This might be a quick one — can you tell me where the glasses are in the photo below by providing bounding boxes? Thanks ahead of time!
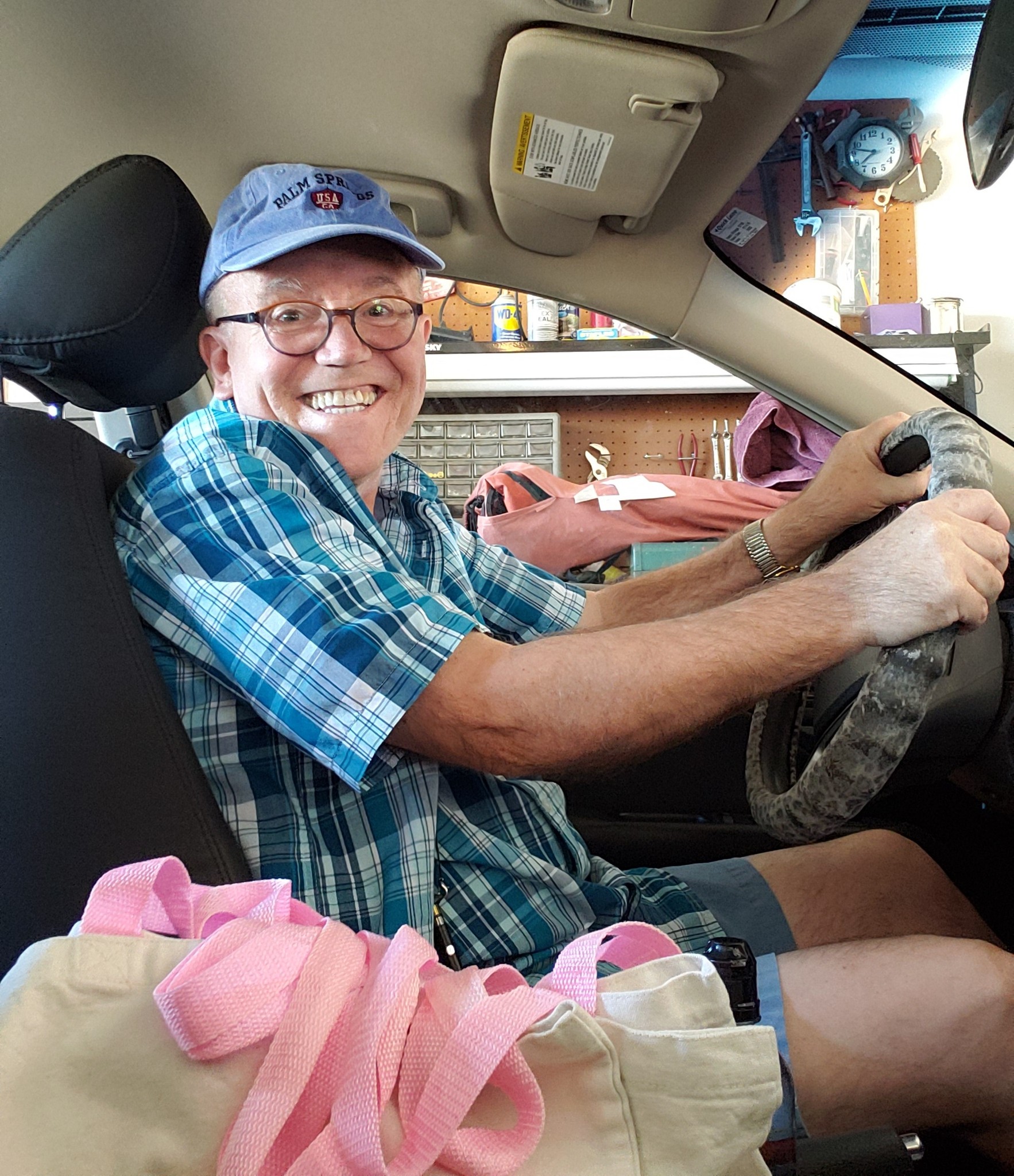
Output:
[214,295,424,357]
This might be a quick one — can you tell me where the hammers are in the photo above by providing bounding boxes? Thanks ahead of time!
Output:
[794,110,839,202]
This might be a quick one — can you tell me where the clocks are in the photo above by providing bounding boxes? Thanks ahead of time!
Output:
[835,116,913,193]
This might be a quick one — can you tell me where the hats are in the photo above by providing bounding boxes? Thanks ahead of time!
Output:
[199,163,446,308]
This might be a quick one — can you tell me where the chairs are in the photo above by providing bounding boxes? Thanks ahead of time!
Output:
[0,155,250,980]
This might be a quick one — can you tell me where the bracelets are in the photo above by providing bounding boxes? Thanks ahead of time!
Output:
[744,518,801,585]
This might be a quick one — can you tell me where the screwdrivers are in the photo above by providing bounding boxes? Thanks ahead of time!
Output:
[910,134,927,194]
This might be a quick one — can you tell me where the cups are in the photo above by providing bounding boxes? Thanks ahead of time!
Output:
[782,278,841,329]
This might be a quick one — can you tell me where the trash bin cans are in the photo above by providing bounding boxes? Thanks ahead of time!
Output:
[631,542,727,579]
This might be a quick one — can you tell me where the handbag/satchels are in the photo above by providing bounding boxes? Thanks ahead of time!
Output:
[0,856,784,1175]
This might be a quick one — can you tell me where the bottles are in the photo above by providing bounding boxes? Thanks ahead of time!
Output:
[703,936,798,1175]
[527,293,613,343]
[490,288,521,342]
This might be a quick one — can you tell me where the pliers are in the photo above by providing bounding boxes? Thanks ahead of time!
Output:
[678,434,698,477]
[899,127,940,185]
[811,179,861,206]
[963,0,1013,189]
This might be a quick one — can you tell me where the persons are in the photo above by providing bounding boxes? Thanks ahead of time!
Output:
[110,159,1012,1175]
